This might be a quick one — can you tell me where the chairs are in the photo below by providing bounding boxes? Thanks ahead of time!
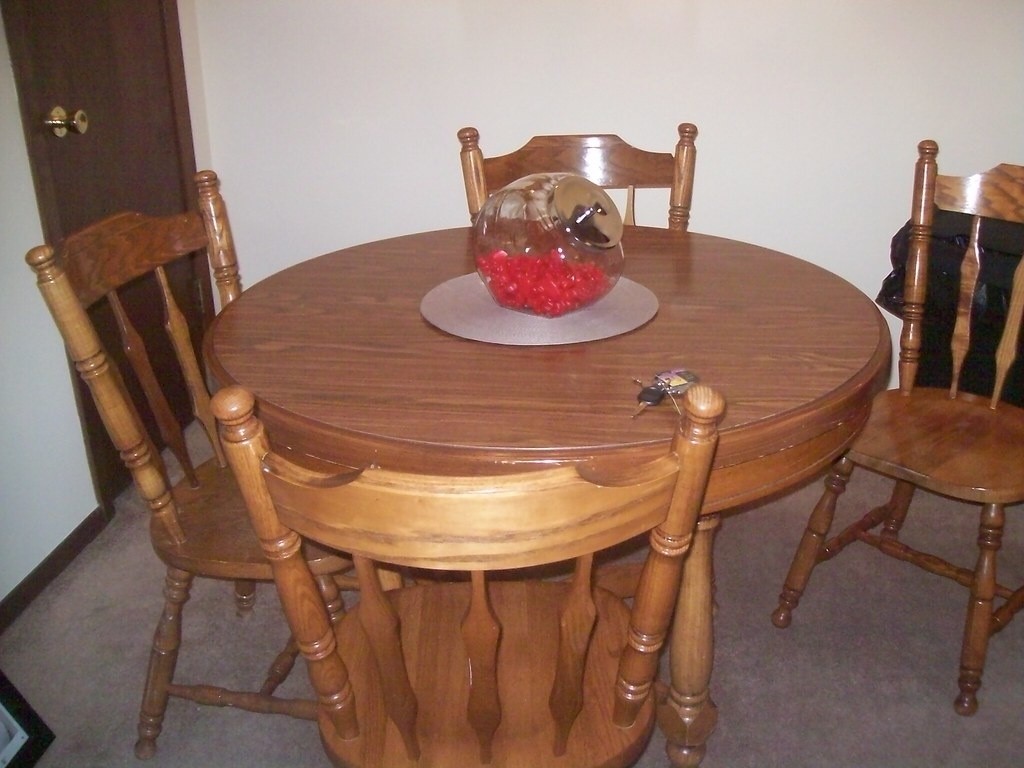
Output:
[770,140,1024,717]
[207,385,727,768]
[458,122,699,232]
[24,169,357,768]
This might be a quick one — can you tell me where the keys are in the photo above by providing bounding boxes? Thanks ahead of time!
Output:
[628,371,682,419]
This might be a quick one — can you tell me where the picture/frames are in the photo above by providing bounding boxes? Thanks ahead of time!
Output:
[0,669,56,768]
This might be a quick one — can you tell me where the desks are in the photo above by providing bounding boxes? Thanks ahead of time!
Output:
[199,225,892,598]
